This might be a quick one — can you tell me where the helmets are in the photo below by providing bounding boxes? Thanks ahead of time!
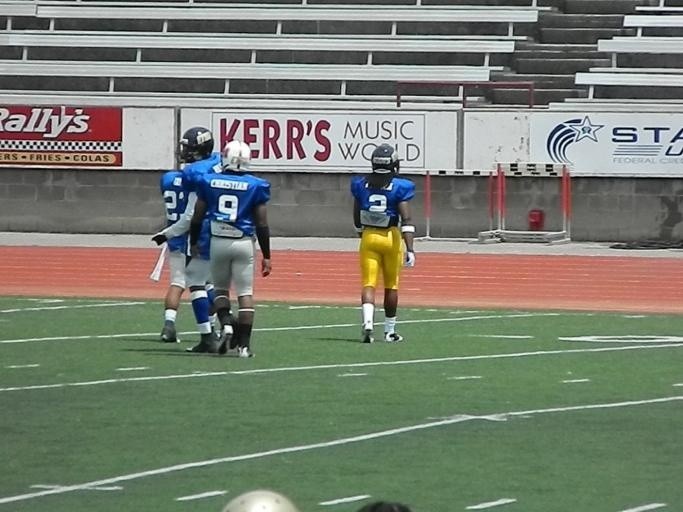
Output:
[371,144,399,176]
[221,141,251,173]
[180,127,213,161]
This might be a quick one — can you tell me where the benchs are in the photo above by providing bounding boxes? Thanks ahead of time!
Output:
[0,1,683,111]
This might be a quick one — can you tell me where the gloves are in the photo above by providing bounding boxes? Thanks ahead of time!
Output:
[405,251,415,267]
[151,232,167,245]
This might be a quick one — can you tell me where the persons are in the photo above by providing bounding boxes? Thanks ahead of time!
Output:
[150,126,241,352]
[190,141,271,359]
[159,170,217,338]
[349,143,416,344]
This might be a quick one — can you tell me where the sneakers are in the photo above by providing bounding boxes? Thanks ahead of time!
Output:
[161,320,255,358]
[385,332,403,342]
[362,321,375,343]
